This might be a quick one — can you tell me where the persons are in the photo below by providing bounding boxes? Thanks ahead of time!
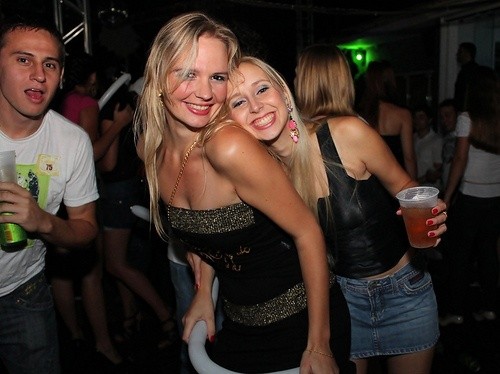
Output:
[50,52,178,374]
[225,45,448,374]
[439,98,463,194]
[453,42,481,113]
[411,105,444,199]
[353,60,418,184]
[132,13,357,374]
[0,18,100,374]
[440,66,500,327]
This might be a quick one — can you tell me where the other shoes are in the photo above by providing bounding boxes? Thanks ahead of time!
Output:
[440,316,464,327]
[472,311,497,323]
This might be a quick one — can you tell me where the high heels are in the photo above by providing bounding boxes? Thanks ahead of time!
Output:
[95,350,127,374]
[71,337,88,353]
[151,325,180,356]
[115,309,144,343]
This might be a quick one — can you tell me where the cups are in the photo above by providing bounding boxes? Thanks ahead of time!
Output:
[395,186,440,249]
[0,150,18,185]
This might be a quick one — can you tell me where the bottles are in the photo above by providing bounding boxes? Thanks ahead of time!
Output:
[0,201,28,253]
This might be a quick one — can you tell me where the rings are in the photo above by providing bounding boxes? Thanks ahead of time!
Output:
[442,211,448,217]
